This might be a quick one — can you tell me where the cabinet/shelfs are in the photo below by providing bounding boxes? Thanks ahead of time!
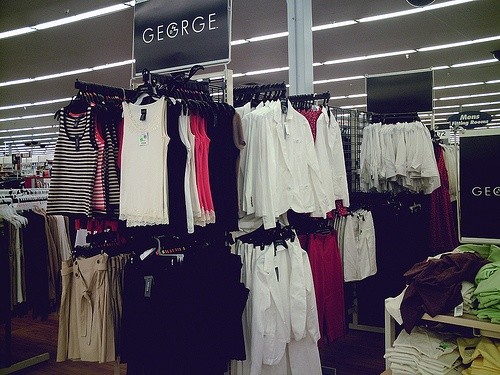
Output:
[382,296,500,375]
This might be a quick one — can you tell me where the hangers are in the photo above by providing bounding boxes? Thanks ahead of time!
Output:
[67,190,441,265]
[365,113,459,151]
[0,189,69,230]
[53,70,337,122]
